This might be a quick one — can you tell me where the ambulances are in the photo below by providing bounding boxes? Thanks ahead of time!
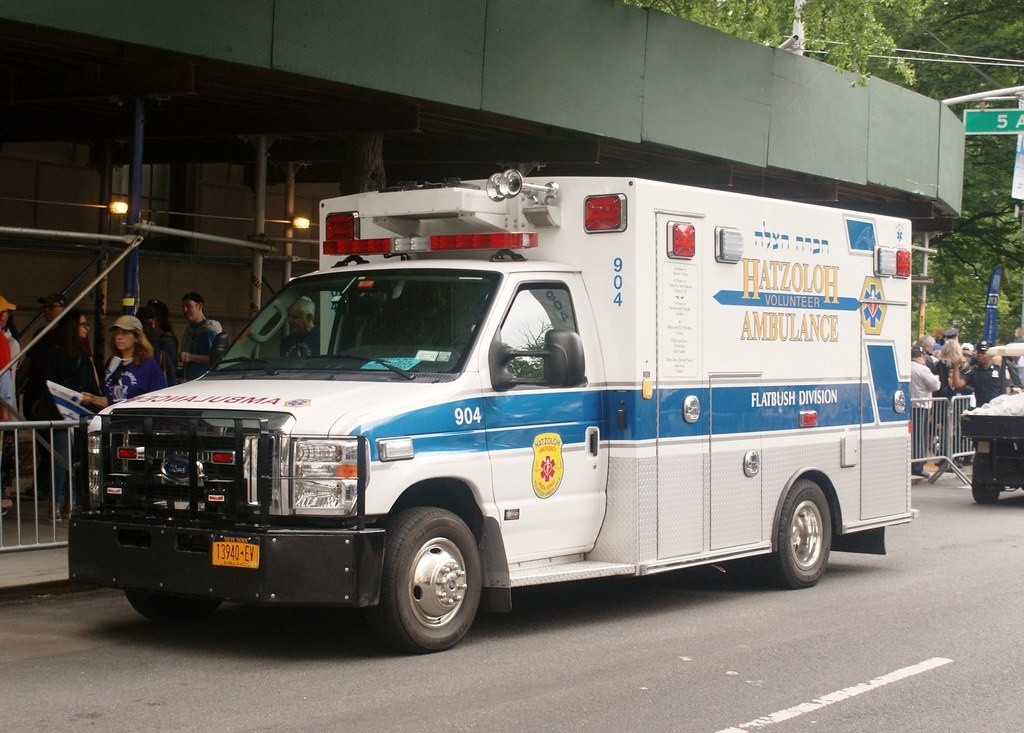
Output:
[66,167,920,661]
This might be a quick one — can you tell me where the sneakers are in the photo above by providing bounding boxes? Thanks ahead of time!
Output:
[24,487,49,499]
[2,496,9,516]
[62,511,69,519]
[48,513,62,523]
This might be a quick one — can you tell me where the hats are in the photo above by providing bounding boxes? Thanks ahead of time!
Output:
[944,329,959,337]
[0,295,16,314]
[183,292,204,304]
[977,341,992,350]
[935,328,943,335]
[37,294,66,306]
[108,315,143,332]
[962,343,974,350]
[911,345,926,355]
[147,299,168,320]
[932,344,941,350]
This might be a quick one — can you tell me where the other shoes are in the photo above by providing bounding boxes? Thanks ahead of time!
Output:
[912,474,929,485]
[935,456,972,471]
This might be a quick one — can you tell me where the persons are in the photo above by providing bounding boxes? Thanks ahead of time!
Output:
[278,295,321,358]
[908,324,1024,477]
[0,292,224,525]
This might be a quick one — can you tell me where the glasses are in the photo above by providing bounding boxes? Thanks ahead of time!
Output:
[79,322,89,326]
[40,305,63,310]
